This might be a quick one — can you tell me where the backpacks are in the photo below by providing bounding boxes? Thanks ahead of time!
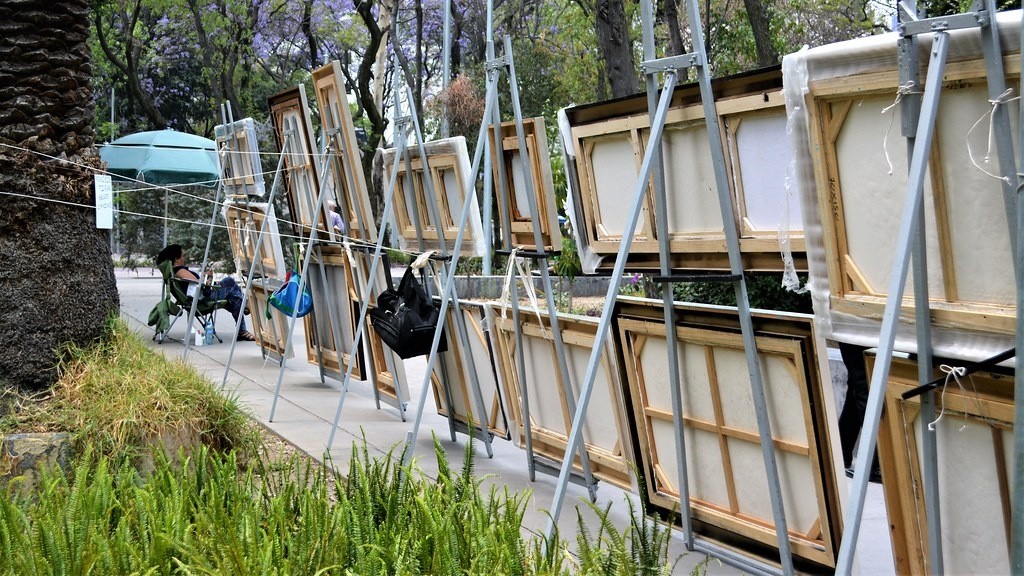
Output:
[370,253,448,360]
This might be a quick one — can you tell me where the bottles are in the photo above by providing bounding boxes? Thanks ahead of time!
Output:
[205,268,213,286]
[205,322,213,344]
[195,329,203,346]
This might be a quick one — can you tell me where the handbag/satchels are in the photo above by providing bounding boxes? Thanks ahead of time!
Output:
[265,243,314,320]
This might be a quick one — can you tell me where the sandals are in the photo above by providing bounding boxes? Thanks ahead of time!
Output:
[244,307,250,315]
[237,330,256,341]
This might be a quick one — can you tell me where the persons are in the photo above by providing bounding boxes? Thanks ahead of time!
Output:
[837,340,882,484]
[156,244,256,341]
[327,200,344,243]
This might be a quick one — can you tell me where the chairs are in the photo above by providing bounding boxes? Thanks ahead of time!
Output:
[157,260,226,344]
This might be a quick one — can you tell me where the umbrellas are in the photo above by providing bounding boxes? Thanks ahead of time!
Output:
[99,126,223,248]
